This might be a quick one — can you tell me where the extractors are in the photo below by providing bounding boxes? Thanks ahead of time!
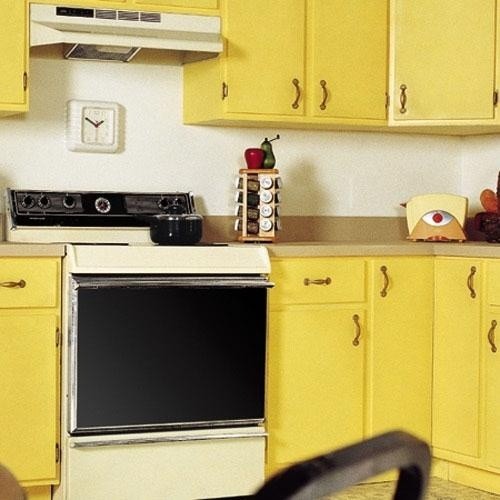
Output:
[29,3,225,64]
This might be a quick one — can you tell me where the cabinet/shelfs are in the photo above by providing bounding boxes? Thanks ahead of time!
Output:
[367,257,433,459]
[267,257,367,466]
[482,258,500,475]
[0,0,30,119]
[432,256,483,472]
[182,0,390,133]
[390,0,500,137]
[0,258,61,488]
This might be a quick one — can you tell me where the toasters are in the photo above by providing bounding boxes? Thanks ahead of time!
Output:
[400,193,469,242]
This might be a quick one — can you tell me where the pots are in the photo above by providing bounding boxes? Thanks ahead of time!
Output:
[146,205,204,243]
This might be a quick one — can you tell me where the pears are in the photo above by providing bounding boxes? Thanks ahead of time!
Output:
[260,134,280,169]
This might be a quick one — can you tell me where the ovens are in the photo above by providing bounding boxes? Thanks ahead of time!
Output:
[67,272,274,438]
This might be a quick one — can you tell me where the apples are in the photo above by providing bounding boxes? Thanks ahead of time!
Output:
[245,149,265,169]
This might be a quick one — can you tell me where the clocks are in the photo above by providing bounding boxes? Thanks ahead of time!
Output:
[67,99,121,153]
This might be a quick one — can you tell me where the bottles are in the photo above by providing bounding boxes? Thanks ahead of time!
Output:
[235,176,259,237]
